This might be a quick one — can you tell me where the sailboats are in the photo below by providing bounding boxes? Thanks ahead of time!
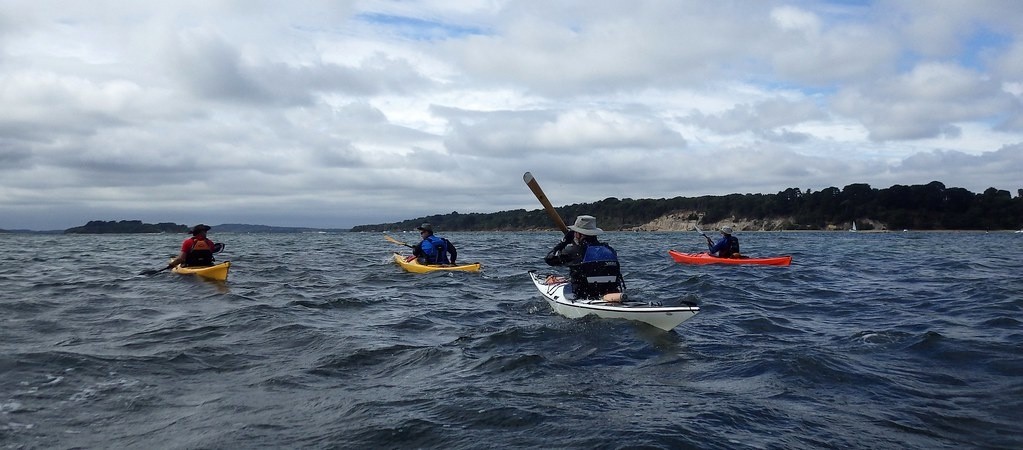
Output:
[849,221,857,233]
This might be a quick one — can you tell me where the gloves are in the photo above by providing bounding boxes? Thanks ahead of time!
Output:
[561,230,573,244]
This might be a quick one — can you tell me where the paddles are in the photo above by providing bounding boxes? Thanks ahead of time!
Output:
[384,235,416,249]
[139,242,225,277]
[522,172,577,245]
[693,224,716,245]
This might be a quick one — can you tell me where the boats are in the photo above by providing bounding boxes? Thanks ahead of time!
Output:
[393,251,480,273]
[168,258,230,281]
[529,272,701,332]
[668,250,792,267]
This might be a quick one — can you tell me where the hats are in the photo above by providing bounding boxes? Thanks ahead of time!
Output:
[718,226,733,234]
[567,215,604,236]
[189,225,211,234]
[417,223,431,231]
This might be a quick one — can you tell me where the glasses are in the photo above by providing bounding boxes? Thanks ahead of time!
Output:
[420,231,425,235]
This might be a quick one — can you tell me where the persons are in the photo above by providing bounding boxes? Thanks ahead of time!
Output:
[411,223,457,264]
[168,224,217,268]
[707,225,740,257]
[544,215,621,299]
